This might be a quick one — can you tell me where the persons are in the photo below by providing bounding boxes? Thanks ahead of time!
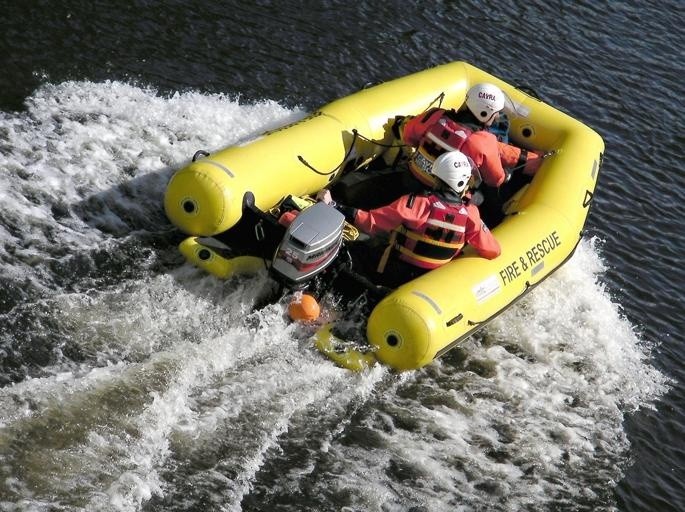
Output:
[316,151,502,285]
[400,83,518,197]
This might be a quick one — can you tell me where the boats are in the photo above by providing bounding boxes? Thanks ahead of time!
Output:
[164,59,606,375]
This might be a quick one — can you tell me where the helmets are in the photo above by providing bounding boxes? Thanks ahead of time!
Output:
[431,150,472,194]
[464,82,507,123]
